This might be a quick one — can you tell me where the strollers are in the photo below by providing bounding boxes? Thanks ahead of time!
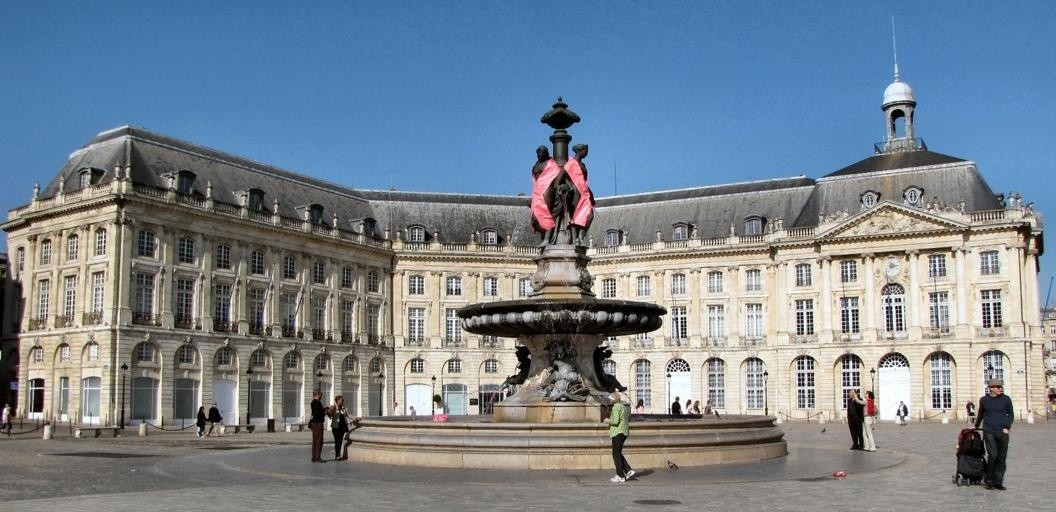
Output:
[951,428,987,485]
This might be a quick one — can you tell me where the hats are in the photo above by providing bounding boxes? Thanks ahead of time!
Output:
[608,392,620,400]
[986,378,1004,387]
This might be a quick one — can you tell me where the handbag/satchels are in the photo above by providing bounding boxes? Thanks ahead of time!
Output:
[956,428,987,455]
[307,415,314,429]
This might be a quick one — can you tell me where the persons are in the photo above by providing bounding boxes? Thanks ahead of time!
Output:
[969,379,1014,490]
[196,401,224,438]
[965,399,975,423]
[0,403,15,439]
[393,402,402,417]
[672,396,714,415]
[847,389,876,452]
[529,144,596,249]
[896,400,908,426]
[307,390,362,463]
[604,393,636,482]
[408,406,417,416]
[636,398,645,414]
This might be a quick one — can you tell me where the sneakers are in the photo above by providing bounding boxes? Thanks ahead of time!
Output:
[311,456,344,463]
[983,483,1007,491]
[626,470,637,481]
[609,474,627,483]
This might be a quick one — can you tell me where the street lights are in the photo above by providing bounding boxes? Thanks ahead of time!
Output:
[120,363,128,429]
[431,375,436,415]
[246,367,253,430]
[378,372,384,415]
[666,372,672,413]
[317,371,323,390]
[987,364,994,380]
[870,368,876,393]
[763,370,768,414]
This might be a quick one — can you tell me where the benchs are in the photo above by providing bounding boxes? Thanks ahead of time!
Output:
[286,422,308,432]
[74,426,121,439]
[220,424,255,433]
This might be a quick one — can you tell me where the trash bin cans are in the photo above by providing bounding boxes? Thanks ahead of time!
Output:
[268,419,274,432]
